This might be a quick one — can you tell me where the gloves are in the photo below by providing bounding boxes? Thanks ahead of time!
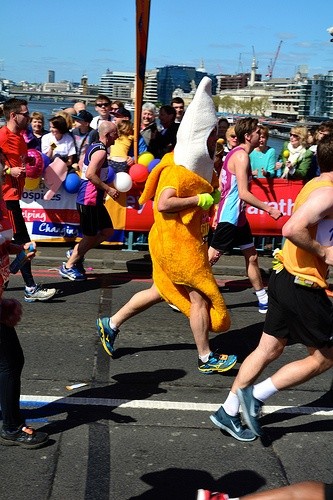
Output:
[211,189,221,204]
[197,193,214,211]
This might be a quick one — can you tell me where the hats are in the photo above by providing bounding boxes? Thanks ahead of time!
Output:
[72,110,93,123]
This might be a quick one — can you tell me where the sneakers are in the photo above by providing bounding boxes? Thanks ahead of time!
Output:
[65,249,93,271]
[258,301,270,313]
[25,282,57,302]
[198,349,238,375]
[0,423,50,449]
[97,317,119,357]
[59,260,88,281]
[237,383,263,437]
[209,405,258,442]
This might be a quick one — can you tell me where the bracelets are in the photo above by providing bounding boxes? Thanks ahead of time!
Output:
[6,168,11,175]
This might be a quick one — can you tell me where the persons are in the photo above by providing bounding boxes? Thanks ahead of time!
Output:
[0,74,333,500]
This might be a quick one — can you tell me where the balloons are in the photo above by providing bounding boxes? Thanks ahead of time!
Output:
[147,159,160,173]
[42,153,49,167]
[25,149,43,179]
[113,172,132,192]
[64,172,81,193]
[130,164,148,182]
[138,152,154,167]
[106,166,114,184]
[23,177,41,191]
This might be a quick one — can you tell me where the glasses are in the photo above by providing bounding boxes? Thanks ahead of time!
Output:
[15,112,29,116]
[230,135,237,138]
[96,103,110,107]
[290,133,299,138]
